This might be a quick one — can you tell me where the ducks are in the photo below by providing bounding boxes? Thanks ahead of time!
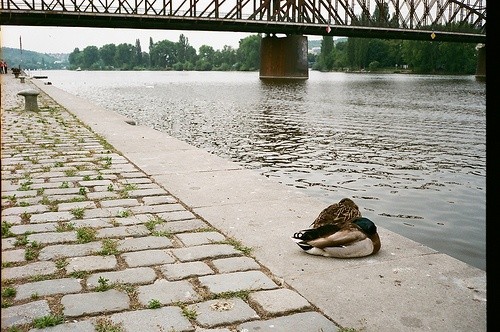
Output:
[291,217,382,259]
[308,198,363,230]
